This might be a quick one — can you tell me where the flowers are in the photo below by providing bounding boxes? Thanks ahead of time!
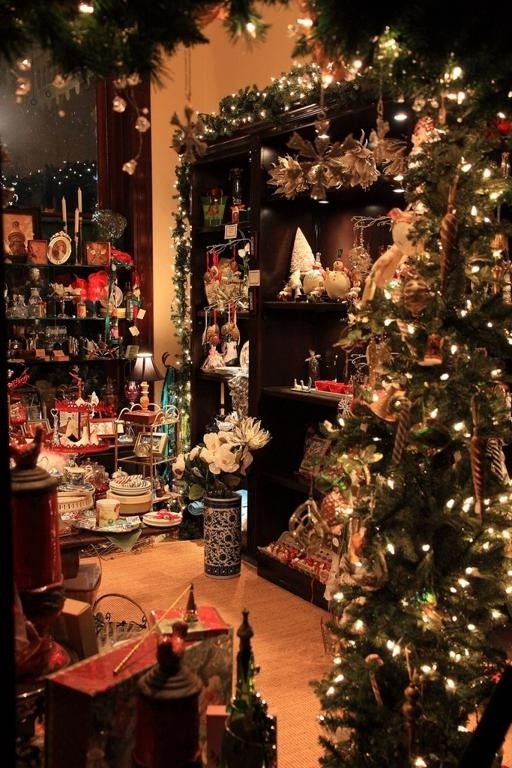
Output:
[167,407,274,495]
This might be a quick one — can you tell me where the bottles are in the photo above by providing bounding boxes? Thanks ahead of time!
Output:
[10,287,48,319]
[77,303,86,318]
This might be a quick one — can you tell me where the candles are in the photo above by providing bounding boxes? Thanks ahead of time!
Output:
[77,187,83,224]
[73,207,79,241]
[61,195,67,233]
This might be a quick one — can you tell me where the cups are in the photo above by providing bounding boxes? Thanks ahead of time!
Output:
[96,499,121,528]
[64,465,93,489]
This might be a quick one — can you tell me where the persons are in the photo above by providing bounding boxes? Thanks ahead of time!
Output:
[52,243,66,261]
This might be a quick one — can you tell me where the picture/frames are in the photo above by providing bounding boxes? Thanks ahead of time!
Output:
[83,240,111,267]
[45,230,72,265]
[132,431,170,457]
[26,239,48,265]
[0,207,41,263]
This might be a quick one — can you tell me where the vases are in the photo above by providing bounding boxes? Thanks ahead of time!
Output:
[203,493,244,578]
[10,286,47,320]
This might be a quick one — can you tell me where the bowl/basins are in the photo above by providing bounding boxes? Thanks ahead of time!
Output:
[314,380,353,396]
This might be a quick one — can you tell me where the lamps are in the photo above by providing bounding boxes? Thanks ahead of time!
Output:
[126,348,165,415]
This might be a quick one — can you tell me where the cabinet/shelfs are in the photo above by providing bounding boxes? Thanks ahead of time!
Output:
[0,261,126,415]
[256,96,398,610]
[186,123,260,450]
[113,402,182,492]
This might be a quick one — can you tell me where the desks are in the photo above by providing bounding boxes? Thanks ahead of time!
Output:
[57,512,180,580]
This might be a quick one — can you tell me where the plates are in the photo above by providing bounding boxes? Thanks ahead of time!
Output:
[143,511,182,527]
[60,510,141,533]
[109,479,152,496]
[239,339,249,375]
